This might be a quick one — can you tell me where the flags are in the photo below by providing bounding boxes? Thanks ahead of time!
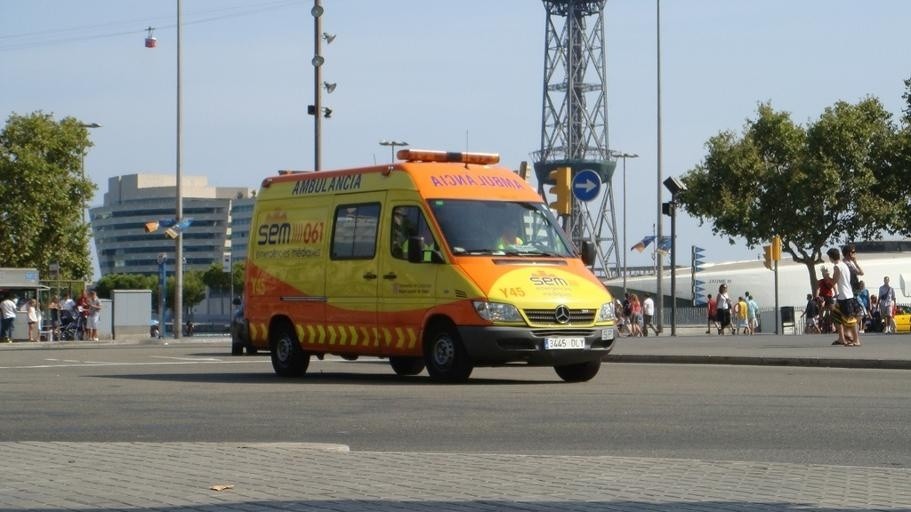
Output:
[630,235,658,254]
[145,218,176,233]
[163,218,194,239]
[653,236,676,256]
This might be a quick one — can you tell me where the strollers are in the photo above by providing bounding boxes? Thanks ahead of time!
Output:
[40,297,87,341]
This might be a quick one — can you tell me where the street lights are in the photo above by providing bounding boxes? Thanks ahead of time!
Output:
[660,175,687,336]
[380,141,409,163]
[182,257,187,322]
[614,152,639,304]
[307,0,336,172]
[157,252,167,340]
[76,120,103,289]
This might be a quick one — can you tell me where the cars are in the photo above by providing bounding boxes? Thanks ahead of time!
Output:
[230,296,257,354]
[864,306,911,335]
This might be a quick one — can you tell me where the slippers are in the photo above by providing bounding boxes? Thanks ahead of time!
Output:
[832,340,861,346]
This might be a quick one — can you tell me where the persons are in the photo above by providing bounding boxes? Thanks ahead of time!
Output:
[497,219,523,250]
[403,214,434,262]
[800,244,897,346]
[705,284,760,336]
[615,293,659,337]
[0,289,102,343]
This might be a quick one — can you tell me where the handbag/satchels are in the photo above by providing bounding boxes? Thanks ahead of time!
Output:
[880,294,887,300]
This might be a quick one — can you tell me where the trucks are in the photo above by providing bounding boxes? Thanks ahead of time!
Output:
[243,149,618,383]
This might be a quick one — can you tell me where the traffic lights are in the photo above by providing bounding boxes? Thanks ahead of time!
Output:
[548,166,566,217]
[762,245,772,269]
